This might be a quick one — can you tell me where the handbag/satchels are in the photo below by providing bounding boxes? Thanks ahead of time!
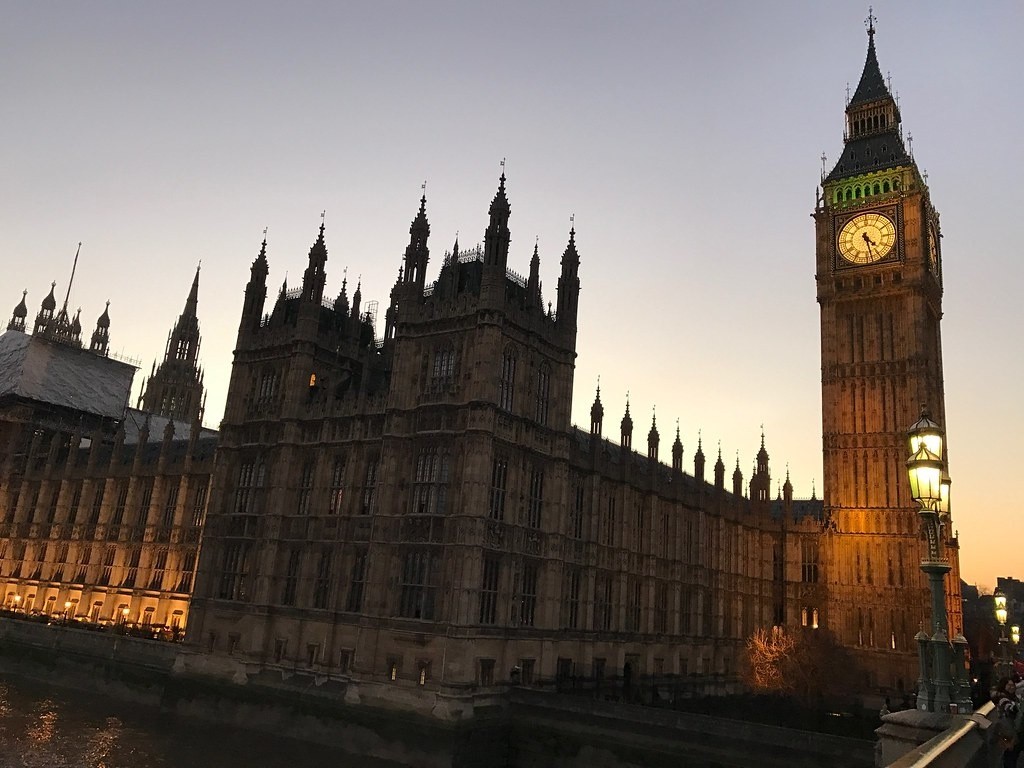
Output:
[998,730,1016,752]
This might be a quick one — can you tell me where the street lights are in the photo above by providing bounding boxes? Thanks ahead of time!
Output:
[13,595,21,613]
[902,398,974,716]
[992,584,1013,685]
[1009,624,1022,662]
[62,602,72,626]
[122,608,129,635]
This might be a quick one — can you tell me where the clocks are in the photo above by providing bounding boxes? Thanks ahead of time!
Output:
[836,213,896,264]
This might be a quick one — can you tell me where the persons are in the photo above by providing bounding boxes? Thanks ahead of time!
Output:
[989,676,1023,768]
[879,704,890,718]
